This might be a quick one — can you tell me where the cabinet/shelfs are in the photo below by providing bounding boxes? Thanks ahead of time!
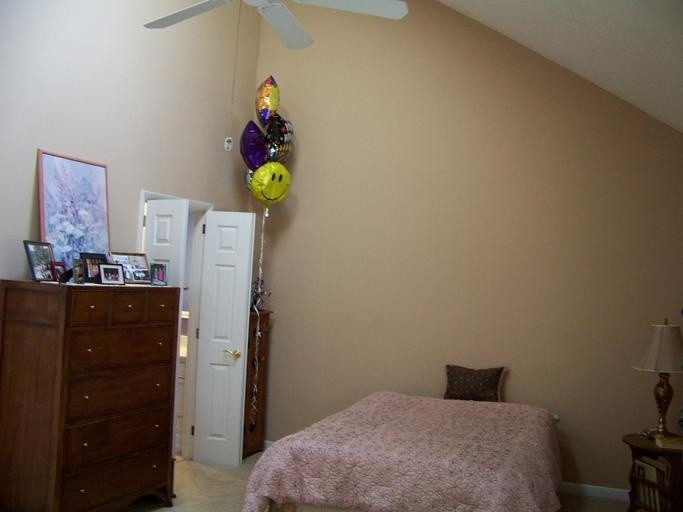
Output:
[0,278,180,512]
[243,309,273,459]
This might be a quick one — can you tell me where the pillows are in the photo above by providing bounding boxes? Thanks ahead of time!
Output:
[441,363,508,401]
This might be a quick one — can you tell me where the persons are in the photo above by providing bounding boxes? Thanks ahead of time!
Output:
[106,272,121,281]
[153,268,158,279]
[158,266,164,281]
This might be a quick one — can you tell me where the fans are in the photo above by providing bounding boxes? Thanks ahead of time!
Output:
[141,0,409,49]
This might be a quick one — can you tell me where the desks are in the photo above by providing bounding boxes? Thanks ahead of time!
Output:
[623,434,683,512]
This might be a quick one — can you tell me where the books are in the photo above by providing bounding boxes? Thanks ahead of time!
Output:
[655,434,683,450]
[634,455,671,512]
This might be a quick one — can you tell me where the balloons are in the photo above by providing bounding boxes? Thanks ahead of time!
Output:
[240,75,295,204]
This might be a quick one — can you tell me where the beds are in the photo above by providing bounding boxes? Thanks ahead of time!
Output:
[242,390,564,512]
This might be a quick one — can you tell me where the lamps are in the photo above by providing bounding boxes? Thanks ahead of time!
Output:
[632,318,683,439]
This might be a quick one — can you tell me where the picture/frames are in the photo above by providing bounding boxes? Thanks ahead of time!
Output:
[150,263,167,285]
[37,148,111,270]
[23,240,58,281]
[80,252,107,282]
[98,263,125,285]
[108,251,151,283]
[54,261,67,282]
